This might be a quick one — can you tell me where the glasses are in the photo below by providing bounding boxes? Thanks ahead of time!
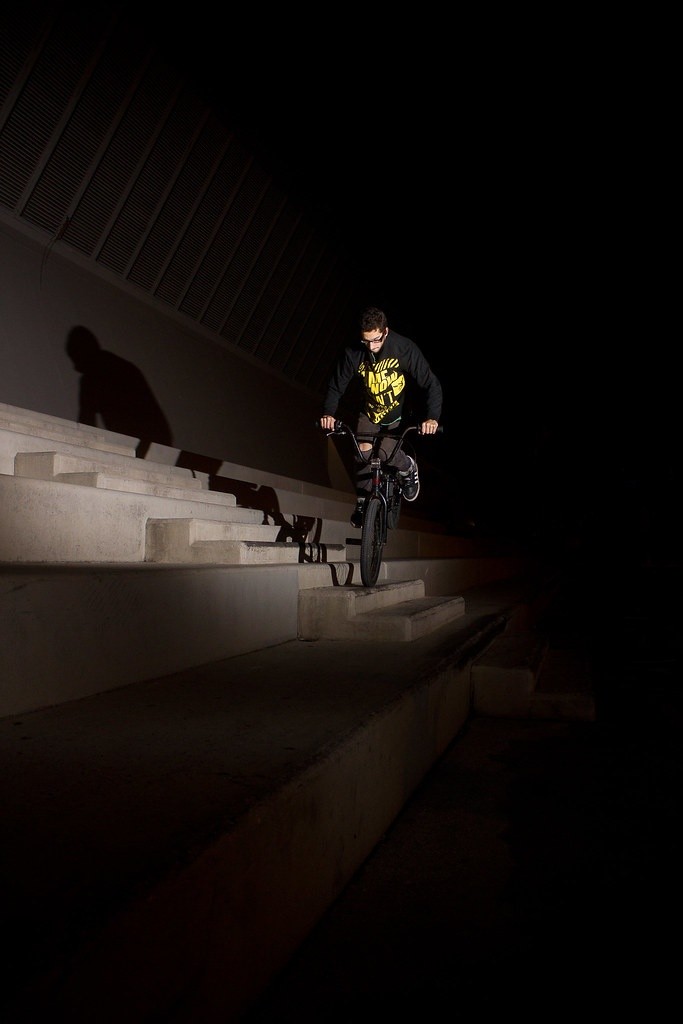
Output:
[361,331,386,344]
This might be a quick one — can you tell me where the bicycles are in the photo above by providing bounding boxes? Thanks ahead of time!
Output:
[310,414,444,592]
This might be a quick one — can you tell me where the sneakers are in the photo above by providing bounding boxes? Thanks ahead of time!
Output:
[399,456,420,501]
[351,498,364,528]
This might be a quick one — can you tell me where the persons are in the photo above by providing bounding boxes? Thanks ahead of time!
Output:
[320,306,444,528]
